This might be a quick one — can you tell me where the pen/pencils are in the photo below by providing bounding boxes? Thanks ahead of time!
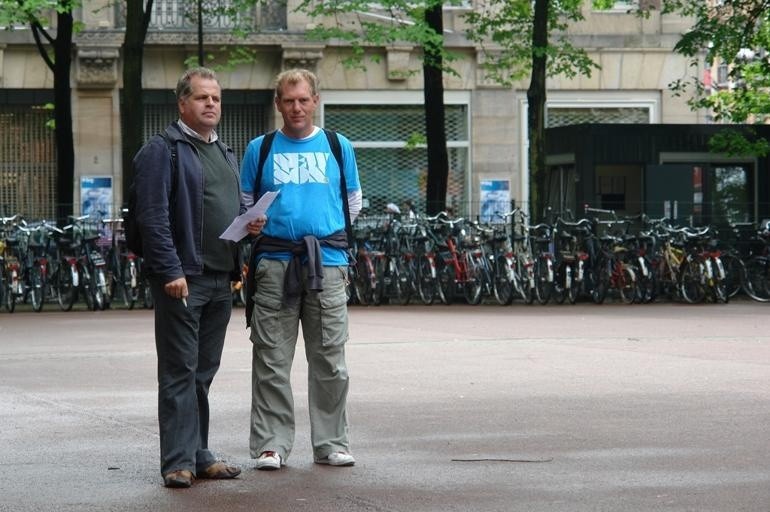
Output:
[182,297,187,308]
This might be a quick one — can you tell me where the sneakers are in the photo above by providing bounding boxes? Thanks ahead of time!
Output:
[258,451,281,470]
[164,471,192,488]
[197,463,241,479]
[315,453,355,466]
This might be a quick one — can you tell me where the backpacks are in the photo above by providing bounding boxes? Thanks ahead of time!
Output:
[125,130,176,257]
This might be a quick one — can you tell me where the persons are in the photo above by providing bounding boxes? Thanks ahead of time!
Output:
[131,67,267,489]
[387,204,402,222]
[404,200,415,221]
[444,207,457,220]
[239,68,363,470]
